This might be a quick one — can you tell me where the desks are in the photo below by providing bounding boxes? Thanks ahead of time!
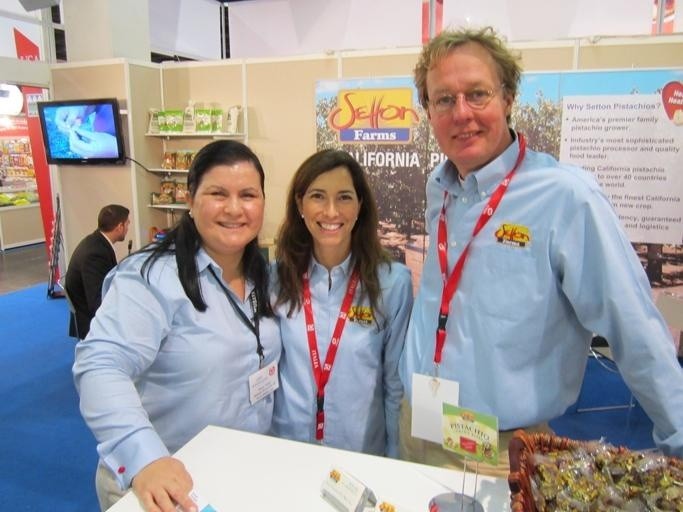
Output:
[107,424,512,512]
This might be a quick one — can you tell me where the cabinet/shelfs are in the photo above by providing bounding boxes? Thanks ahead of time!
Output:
[1,57,56,251]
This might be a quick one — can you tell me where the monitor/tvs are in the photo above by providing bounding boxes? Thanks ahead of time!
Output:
[37,98,125,167]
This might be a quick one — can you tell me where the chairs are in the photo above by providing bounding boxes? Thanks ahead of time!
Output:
[569,291,683,419]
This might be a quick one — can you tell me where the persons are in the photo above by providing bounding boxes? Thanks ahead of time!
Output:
[71,139,277,511]
[399,26,682,474]
[270,148,411,456]
[65,204,131,340]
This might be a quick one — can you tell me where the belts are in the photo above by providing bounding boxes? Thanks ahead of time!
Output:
[498,428,527,433]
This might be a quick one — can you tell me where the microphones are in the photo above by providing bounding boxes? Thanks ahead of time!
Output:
[127,239,133,256]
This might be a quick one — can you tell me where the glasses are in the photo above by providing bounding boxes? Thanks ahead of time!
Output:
[425,83,507,113]
